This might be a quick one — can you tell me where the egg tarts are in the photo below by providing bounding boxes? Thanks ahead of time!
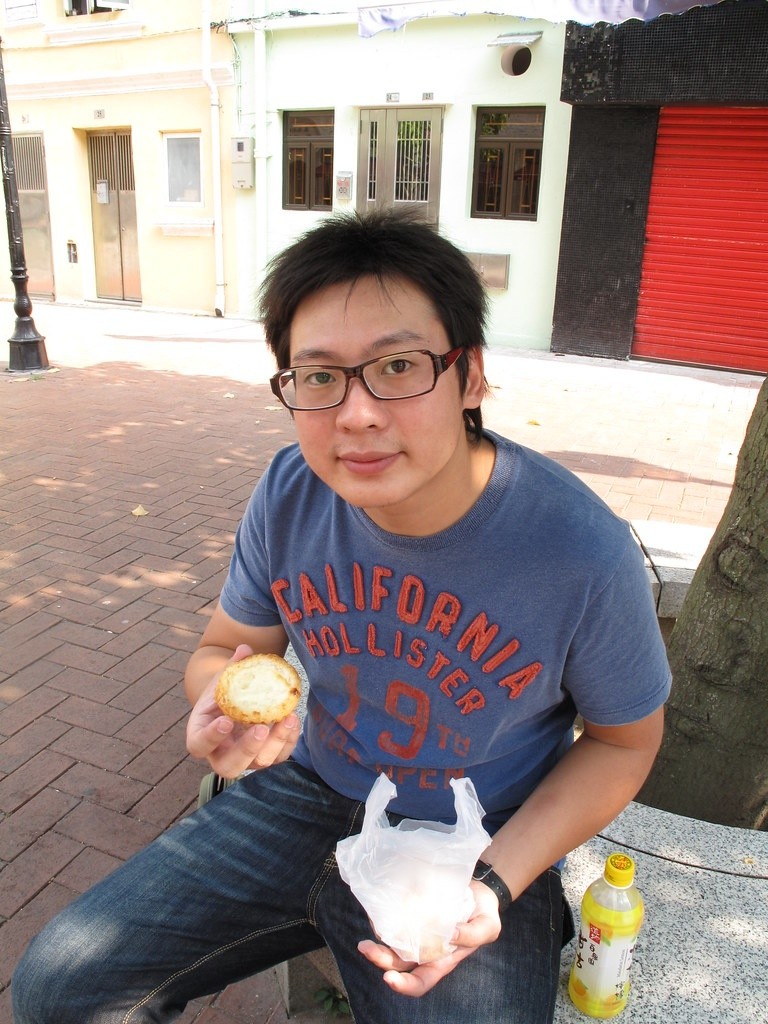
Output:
[216,652,302,724]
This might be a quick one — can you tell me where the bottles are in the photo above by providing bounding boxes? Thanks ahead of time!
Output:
[568,855,644,1018]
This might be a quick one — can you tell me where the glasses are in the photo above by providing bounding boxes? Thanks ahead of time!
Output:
[269,346,463,411]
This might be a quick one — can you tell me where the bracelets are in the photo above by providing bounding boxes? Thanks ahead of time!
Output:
[469,857,513,913]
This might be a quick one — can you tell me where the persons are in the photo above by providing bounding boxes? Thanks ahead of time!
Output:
[5,210,673,1024]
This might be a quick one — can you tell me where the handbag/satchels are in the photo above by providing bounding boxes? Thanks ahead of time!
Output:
[333,771,491,965]
